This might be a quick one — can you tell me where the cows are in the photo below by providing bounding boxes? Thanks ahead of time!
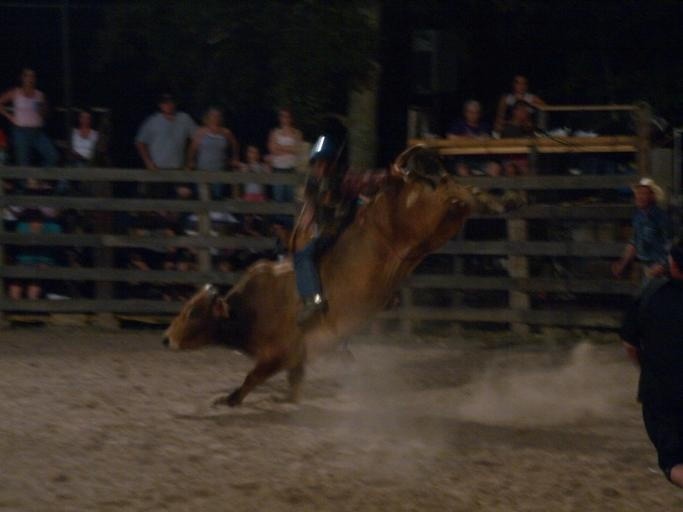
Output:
[161,143,527,408]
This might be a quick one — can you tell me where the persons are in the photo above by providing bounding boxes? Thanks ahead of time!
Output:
[610,176,668,285]
[617,240,682,490]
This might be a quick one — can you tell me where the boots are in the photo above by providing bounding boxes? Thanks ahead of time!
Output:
[296,292,326,325]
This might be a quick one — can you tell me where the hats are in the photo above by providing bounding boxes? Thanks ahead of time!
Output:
[670,239,683,261]
[629,177,665,202]
[157,92,177,103]
[307,133,335,163]
